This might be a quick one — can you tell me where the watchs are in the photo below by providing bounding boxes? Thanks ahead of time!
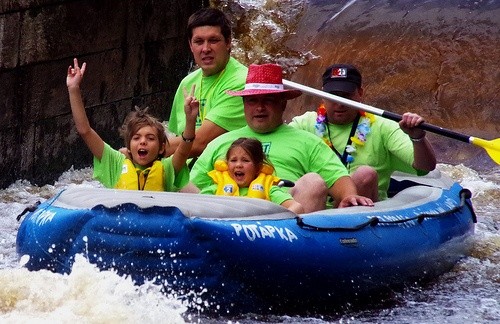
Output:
[181,131,196,143]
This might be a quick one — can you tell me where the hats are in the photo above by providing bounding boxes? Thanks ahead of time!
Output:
[322,64,363,96]
[225,63,303,100]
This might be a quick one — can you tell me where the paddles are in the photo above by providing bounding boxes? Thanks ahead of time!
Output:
[281,78,500,167]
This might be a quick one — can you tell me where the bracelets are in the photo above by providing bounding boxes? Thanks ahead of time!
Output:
[409,133,429,143]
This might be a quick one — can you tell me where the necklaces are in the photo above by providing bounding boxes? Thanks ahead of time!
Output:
[315,101,375,164]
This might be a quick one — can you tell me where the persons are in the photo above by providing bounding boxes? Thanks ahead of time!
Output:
[66,9,437,216]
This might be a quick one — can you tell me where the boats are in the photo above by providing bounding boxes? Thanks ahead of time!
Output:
[16,159,478,317]
